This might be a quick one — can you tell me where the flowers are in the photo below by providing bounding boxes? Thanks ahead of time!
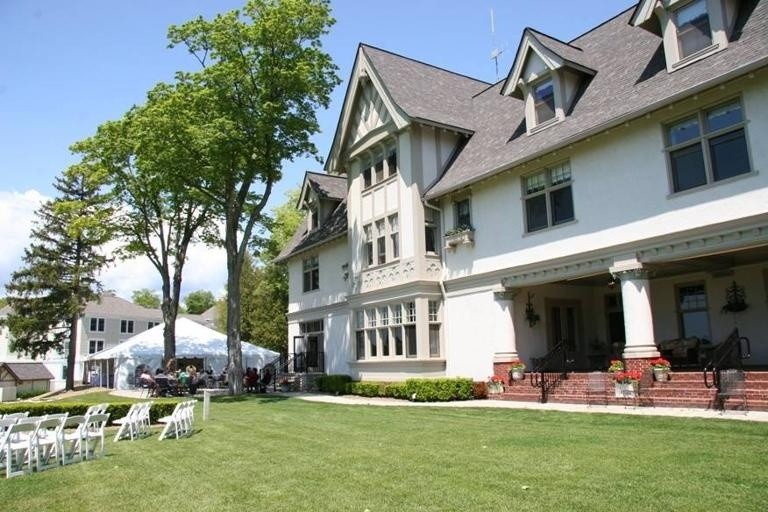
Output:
[486,357,524,386]
[608,358,671,385]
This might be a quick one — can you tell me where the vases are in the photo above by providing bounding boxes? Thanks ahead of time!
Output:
[614,380,638,399]
[488,384,504,393]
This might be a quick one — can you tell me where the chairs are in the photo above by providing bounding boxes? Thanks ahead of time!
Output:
[0,373,215,477]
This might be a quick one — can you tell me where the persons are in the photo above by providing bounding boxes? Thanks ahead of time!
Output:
[138,362,271,398]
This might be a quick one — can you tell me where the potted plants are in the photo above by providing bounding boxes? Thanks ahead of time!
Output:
[443,224,476,248]
